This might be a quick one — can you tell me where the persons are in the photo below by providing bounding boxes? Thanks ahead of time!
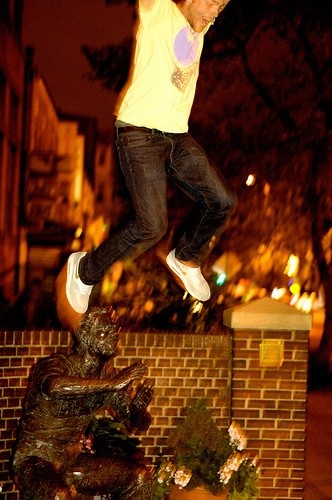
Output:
[65,0,240,311]
[10,300,155,499]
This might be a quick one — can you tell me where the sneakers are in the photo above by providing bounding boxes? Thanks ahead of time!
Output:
[66,252,93,315]
[165,248,211,301]
